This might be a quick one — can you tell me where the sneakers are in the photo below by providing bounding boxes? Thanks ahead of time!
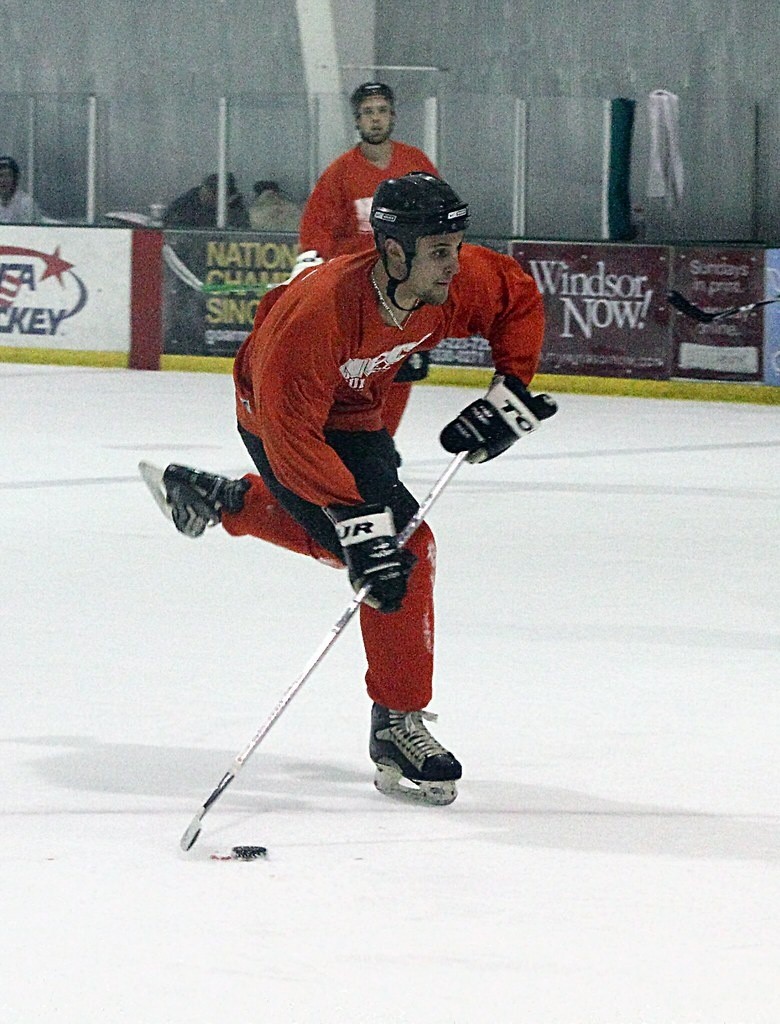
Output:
[138,458,248,538]
[369,701,461,802]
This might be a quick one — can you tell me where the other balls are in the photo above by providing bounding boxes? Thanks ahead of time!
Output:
[232,847,267,861]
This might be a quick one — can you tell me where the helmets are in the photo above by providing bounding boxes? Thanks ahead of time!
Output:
[349,80,394,121]
[369,173,470,264]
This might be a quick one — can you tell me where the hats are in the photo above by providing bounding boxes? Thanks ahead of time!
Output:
[0,154,19,174]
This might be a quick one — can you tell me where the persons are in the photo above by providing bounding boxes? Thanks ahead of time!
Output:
[164,172,548,781]
[163,169,303,230]
[251,83,442,433]
[0,156,45,223]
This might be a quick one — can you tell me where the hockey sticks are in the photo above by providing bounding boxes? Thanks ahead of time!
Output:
[105,212,167,228]
[178,370,506,862]
[161,243,289,292]
[666,289,780,324]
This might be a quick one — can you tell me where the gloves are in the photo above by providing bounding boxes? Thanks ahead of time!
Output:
[440,370,558,463]
[323,504,415,615]
